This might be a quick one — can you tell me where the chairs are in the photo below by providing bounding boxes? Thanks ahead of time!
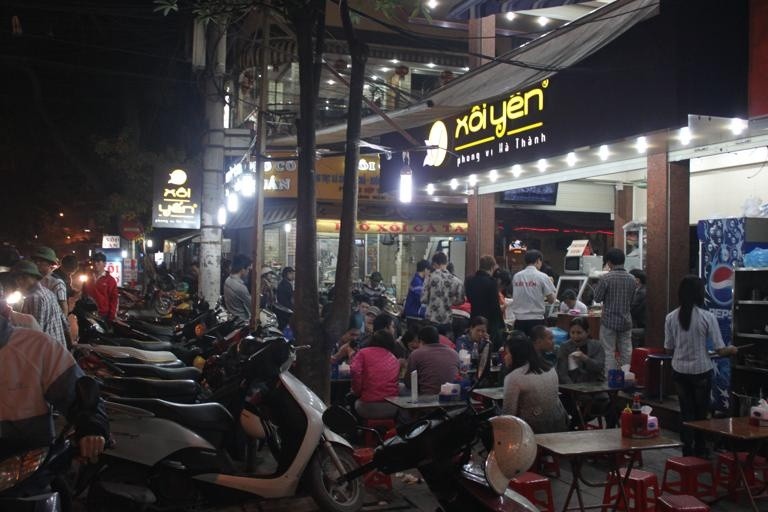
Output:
[452,315,470,338]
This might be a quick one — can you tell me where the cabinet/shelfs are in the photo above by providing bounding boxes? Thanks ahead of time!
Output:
[624,227,648,270]
[730,267,768,418]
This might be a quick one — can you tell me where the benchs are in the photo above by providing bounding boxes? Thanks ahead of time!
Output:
[273,304,293,325]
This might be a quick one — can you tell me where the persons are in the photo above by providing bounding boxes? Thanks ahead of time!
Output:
[560,287,588,314]
[262,267,275,309]
[529,324,555,359]
[501,332,569,435]
[320,252,511,420]
[630,268,646,328]
[593,248,634,376]
[221,260,232,295]
[189,260,199,296]
[278,266,294,309]
[224,254,252,322]
[511,249,556,333]
[556,315,609,430]
[81,253,118,321]
[664,273,738,458]
[0,240,79,461]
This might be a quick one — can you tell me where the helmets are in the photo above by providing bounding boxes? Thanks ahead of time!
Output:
[262,266,273,277]
[239,401,279,440]
[485,415,537,496]
[31,246,58,265]
[8,260,42,282]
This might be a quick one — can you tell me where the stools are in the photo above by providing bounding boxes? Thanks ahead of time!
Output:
[362,419,395,447]
[657,495,710,512]
[532,448,560,477]
[601,468,660,511]
[570,398,603,430]
[661,456,716,498]
[713,453,768,505]
[469,396,486,414]
[508,472,553,512]
[642,353,673,403]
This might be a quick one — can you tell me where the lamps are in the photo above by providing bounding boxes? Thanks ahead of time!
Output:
[399,151,413,204]
[508,238,527,253]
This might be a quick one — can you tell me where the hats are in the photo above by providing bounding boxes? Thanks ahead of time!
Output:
[282,267,295,274]
[91,252,107,261]
[365,305,381,317]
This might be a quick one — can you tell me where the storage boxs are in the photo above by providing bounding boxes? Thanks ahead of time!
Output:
[630,347,678,398]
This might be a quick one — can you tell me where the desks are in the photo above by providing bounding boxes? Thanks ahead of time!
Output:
[268,109,297,130]
[558,309,602,339]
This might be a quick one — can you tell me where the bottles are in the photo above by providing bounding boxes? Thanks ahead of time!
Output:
[459,342,479,370]
[632,393,641,414]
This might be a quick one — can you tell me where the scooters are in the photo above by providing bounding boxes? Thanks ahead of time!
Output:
[67,332,362,512]
[70,292,292,470]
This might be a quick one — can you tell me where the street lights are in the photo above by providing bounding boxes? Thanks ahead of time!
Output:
[246,144,438,330]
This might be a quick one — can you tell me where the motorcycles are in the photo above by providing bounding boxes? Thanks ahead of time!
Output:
[114,271,177,316]
[321,343,543,512]
[0,376,99,512]
